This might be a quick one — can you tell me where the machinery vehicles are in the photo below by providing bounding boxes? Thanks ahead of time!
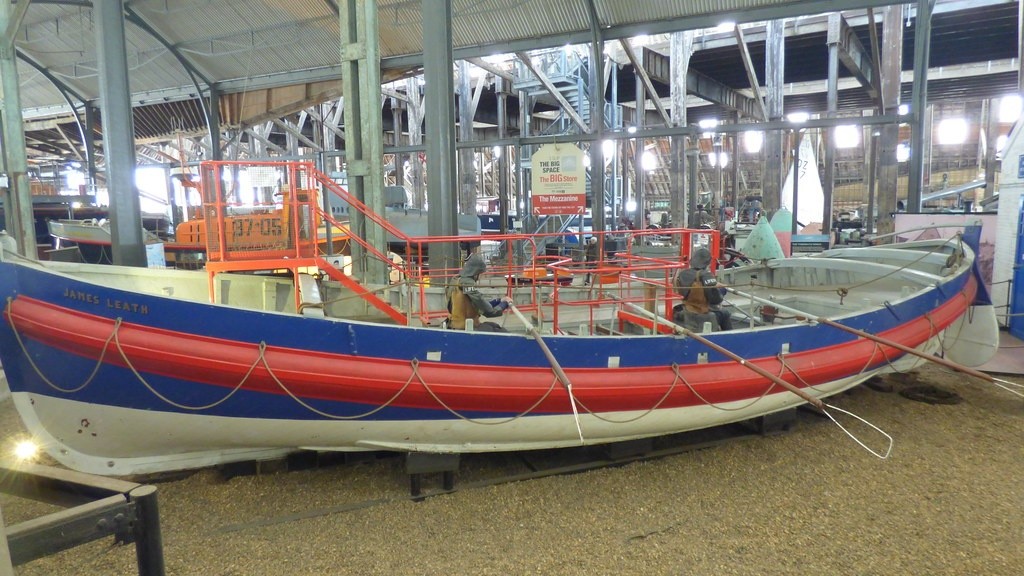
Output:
[175,182,349,257]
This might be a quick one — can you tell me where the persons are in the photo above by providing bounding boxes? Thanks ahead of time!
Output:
[671,249,733,333]
[444,252,513,333]
[582,237,601,286]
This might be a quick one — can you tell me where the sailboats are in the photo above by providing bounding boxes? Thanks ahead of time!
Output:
[782,128,824,235]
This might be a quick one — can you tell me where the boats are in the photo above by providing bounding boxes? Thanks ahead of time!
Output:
[317,185,612,264]
[45,217,165,265]
[0,229,999,475]
